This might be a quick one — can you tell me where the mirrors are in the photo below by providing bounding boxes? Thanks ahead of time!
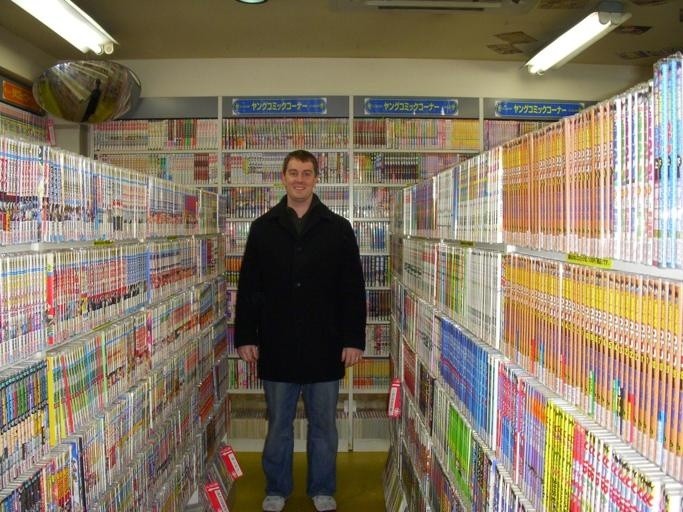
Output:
[32,58,142,125]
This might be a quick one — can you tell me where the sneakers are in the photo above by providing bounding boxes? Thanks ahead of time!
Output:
[262,496,286,511]
[312,495,337,511]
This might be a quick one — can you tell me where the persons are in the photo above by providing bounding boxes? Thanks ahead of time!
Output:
[80,78,102,123]
[233,149,367,511]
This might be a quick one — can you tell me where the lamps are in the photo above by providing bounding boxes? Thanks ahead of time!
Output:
[6,0,118,56]
[520,2,631,80]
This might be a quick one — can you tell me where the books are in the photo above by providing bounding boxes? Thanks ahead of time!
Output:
[301,50,683,510]
[1,100,300,511]
